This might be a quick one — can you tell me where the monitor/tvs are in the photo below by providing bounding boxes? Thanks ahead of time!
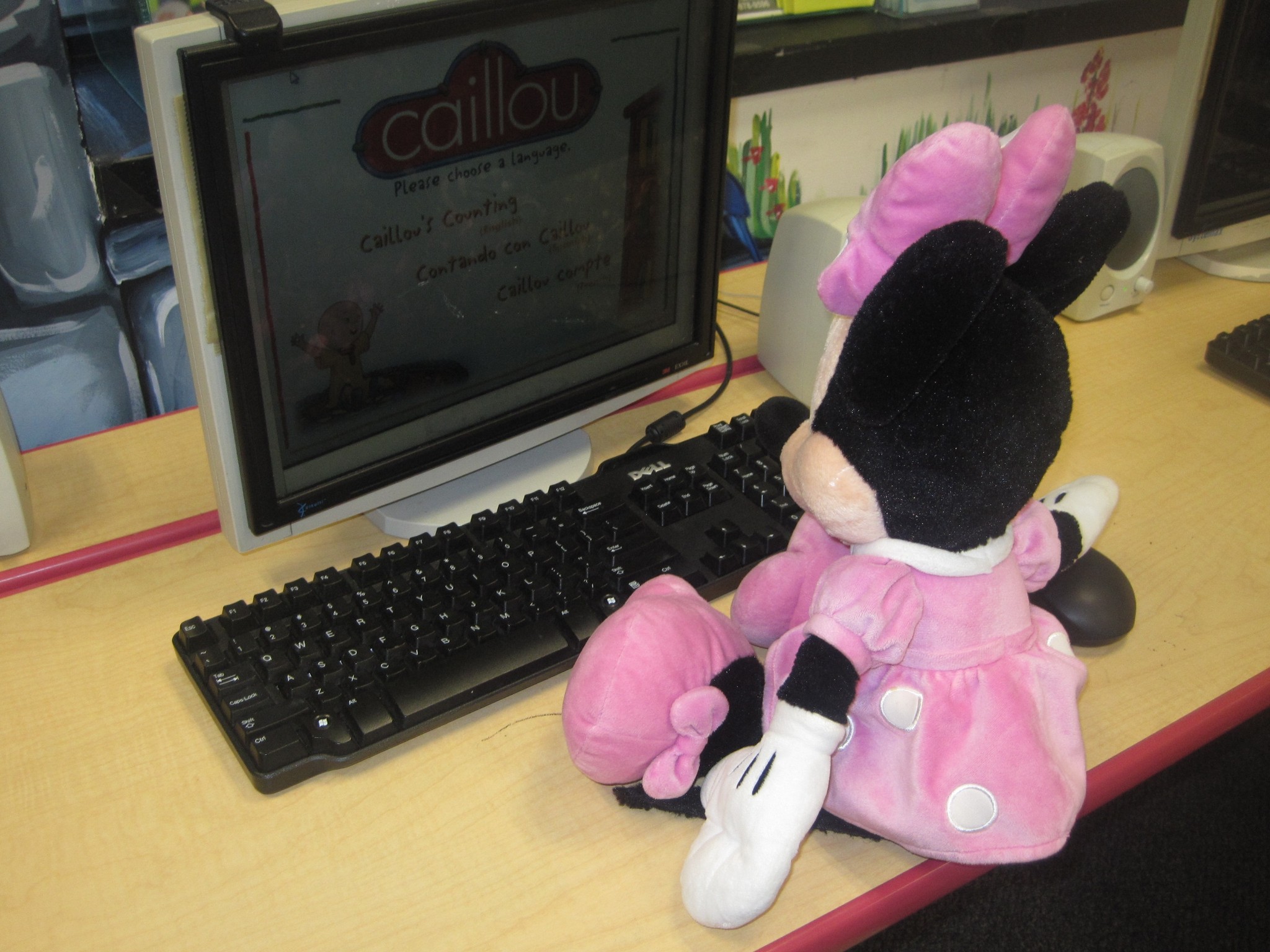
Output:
[1159,0,1270,282]
[133,1,737,554]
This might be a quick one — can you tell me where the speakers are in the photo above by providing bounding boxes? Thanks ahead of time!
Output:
[1059,132,1168,321]
[755,195,871,407]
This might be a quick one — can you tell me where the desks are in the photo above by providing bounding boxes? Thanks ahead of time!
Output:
[0,216,1270,952]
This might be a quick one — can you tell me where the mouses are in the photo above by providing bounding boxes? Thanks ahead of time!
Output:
[1028,546,1136,648]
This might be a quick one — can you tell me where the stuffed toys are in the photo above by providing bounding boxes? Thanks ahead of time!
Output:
[563,104,1133,931]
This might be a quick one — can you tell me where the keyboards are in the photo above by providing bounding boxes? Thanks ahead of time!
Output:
[172,397,809,793]
[1203,313,1270,398]
